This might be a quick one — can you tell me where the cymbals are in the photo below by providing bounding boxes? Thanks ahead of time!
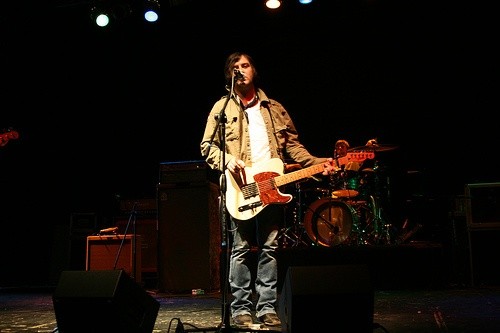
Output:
[347,142,398,152]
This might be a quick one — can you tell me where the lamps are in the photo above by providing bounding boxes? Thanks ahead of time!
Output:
[144,0,160,23]
[90,6,111,28]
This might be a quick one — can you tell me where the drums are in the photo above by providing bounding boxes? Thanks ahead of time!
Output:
[330,189,360,198]
[304,198,353,246]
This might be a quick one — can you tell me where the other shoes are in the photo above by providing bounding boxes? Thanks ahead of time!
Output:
[232,314,253,325]
[259,313,281,325]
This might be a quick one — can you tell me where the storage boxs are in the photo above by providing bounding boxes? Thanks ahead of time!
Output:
[464,182,500,228]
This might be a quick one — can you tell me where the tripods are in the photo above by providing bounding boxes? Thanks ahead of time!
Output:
[276,183,315,248]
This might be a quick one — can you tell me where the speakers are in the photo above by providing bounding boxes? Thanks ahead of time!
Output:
[467,229,500,286]
[86,234,142,283]
[116,219,160,272]
[53,269,160,333]
[464,183,500,226]
[157,183,224,293]
[276,249,375,332]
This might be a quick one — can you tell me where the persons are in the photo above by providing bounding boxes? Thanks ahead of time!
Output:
[199,53,338,328]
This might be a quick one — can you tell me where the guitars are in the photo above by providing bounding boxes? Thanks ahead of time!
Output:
[219,149,375,222]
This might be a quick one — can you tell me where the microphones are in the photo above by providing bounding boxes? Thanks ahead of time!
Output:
[233,68,244,80]
